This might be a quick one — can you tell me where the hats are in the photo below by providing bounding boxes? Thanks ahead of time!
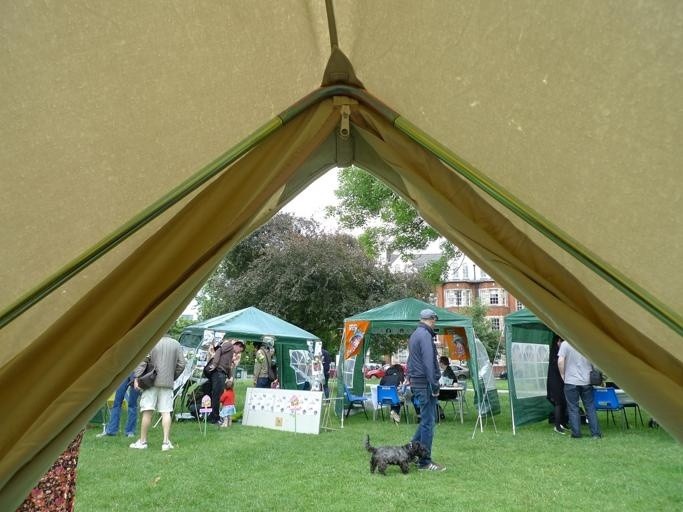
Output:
[421,309,438,320]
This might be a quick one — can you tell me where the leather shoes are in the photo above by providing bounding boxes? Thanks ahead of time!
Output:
[554,426,565,434]
[560,424,571,431]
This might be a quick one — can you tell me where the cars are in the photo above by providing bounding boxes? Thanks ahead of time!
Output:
[366,362,408,380]
[439,364,471,381]
[499,370,509,380]
[327,362,338,379]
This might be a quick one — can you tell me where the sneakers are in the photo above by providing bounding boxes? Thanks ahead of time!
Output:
[418,462,446,472]
[390,411,400,423]
[162,441,173,450]
[129,439,148,449]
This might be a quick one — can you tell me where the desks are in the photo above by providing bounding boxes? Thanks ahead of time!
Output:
[436,385,466,423]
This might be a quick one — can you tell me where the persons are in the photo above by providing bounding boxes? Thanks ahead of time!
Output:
[557,338,603,439]
[546,330,568,435]
[379,364,406,422]
[405,308,446,472]
[183,339,276,428]
[435,355,460,420]
[318,350,333,407]
[130,329,187,451]
[97,371,140,439]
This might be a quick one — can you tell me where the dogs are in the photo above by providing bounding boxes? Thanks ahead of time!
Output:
[366,435,430,476]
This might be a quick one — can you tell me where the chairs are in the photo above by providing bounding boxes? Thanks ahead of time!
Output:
[593,381,643,431]
[344,381,409,424]
[443,380,467,419]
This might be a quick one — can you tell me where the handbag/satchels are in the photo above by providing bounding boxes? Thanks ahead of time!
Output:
[590,370,602,385]
[204,358,216,378]
[127,363,157,390]
[268,368,276,381]
